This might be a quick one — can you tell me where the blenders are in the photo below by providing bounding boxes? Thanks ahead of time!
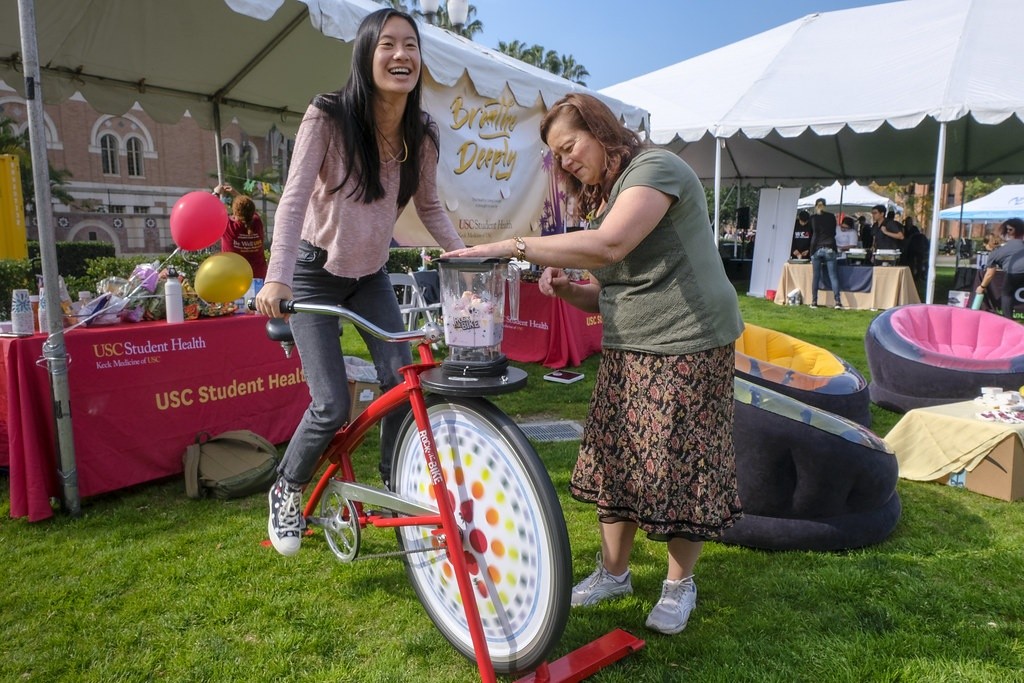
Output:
[432,256,520,377]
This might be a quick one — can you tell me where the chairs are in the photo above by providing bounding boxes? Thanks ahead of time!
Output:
[391,269,443,331]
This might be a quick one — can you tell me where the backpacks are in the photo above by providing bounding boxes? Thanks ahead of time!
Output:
[182,429,281,502]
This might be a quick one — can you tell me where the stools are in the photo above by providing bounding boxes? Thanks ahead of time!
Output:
[734,321,874,430]
[864,304,1024,418]
[714,378,901,551]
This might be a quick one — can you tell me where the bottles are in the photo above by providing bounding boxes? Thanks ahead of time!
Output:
[165,266,184,323]
[79,291,91,309]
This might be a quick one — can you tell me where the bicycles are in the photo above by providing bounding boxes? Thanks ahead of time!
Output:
[262,295,648,683]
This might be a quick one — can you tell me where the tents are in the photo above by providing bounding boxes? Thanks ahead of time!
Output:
[595,0,1024,305]
[0,0,650,519]
[797,180,903,223]
[939,184,1024,239]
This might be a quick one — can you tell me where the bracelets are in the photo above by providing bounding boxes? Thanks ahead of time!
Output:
[980,284,986,289]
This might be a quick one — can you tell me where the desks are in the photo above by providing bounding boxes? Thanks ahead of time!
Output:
[0,313,312,522]
[775,261,922,309]
[497,279,603,368]
[954,267,1024,305]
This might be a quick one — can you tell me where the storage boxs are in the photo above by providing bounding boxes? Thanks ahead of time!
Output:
[948,290,971,307]
[931,433,1024,501]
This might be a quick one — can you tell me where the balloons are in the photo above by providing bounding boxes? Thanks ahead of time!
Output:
[170,191,228,251]
[194,252,253,302]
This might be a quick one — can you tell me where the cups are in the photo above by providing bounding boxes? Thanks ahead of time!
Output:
[38,287,49,334]
[0,322,12,334]
[11,288,34,336]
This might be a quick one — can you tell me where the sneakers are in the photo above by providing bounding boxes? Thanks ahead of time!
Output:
[644,568,697,634]
[570,552,633,609]
[267,475,303,553]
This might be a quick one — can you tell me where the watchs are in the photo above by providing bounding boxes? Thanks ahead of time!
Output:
[515,236,526,262]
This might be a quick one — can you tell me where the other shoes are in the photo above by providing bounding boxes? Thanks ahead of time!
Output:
[834,303,843,310]
[811,300,817,308]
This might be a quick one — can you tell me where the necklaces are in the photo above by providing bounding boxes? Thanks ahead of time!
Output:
[381,128,407,162]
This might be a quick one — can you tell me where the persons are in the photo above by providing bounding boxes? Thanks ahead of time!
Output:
[983,233,1000,251]
[944,235,955,256]
[887,210,904,250]
[791,211,813,259]
[904,216,923,282]
[440,95,744,634]
[255,6,485,556]
[869,205,904,266]
[857,216,874,249]
[836,217,858,252]
[211,185,268,282]
[974,217,1024,293]
[809,202,843,308]
